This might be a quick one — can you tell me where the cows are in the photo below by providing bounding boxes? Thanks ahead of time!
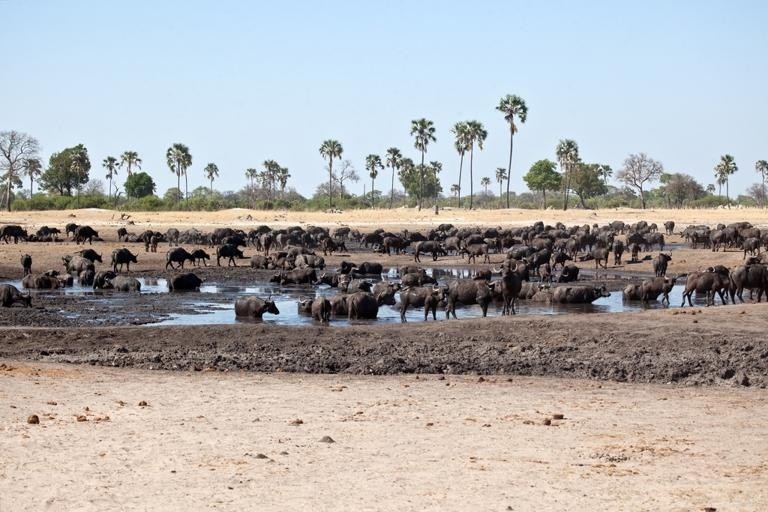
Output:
[0,220,768,323]
